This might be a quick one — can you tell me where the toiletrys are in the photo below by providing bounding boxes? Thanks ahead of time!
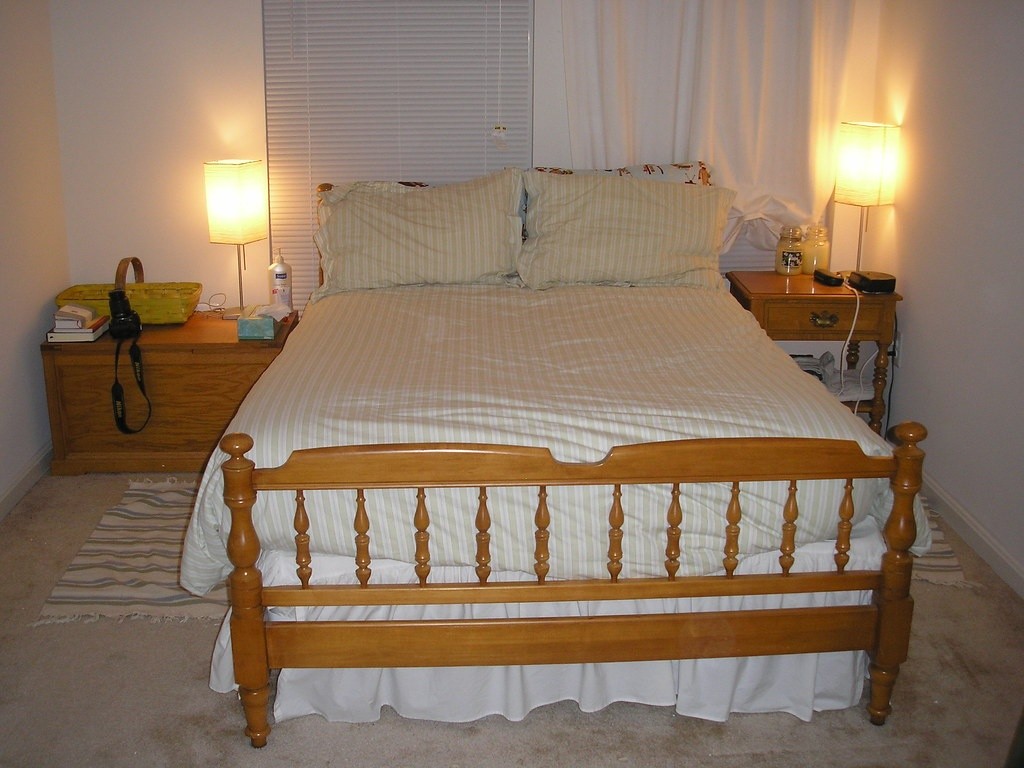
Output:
[267,246,293,310]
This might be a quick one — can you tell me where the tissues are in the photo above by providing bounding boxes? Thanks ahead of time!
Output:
[237,302,291,339]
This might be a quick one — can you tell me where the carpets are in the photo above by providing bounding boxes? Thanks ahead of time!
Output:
[912,493,981,591]
[25,477,229,625]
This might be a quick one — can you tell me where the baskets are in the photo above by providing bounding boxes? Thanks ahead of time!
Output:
[55,257,202,324]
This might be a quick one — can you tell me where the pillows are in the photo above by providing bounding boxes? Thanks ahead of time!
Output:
[311,166,523,303]
[517,169,738,292]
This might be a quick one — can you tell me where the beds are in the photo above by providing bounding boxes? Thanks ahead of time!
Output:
[177,181,930,749]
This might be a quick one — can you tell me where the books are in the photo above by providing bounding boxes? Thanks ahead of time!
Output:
[45,304,110,343]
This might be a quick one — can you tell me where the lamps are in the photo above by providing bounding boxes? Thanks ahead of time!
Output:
[835,121,902,271]
[205,160,268,321]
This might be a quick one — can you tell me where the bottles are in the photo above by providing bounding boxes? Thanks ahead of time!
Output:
[775,225,804,276]
[802,225,831,276]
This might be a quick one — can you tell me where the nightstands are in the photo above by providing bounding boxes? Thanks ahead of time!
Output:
[40,310,299,476]
[726,270,903,435]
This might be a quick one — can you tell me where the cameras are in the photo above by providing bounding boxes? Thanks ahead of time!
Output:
[108,288,142,339]
